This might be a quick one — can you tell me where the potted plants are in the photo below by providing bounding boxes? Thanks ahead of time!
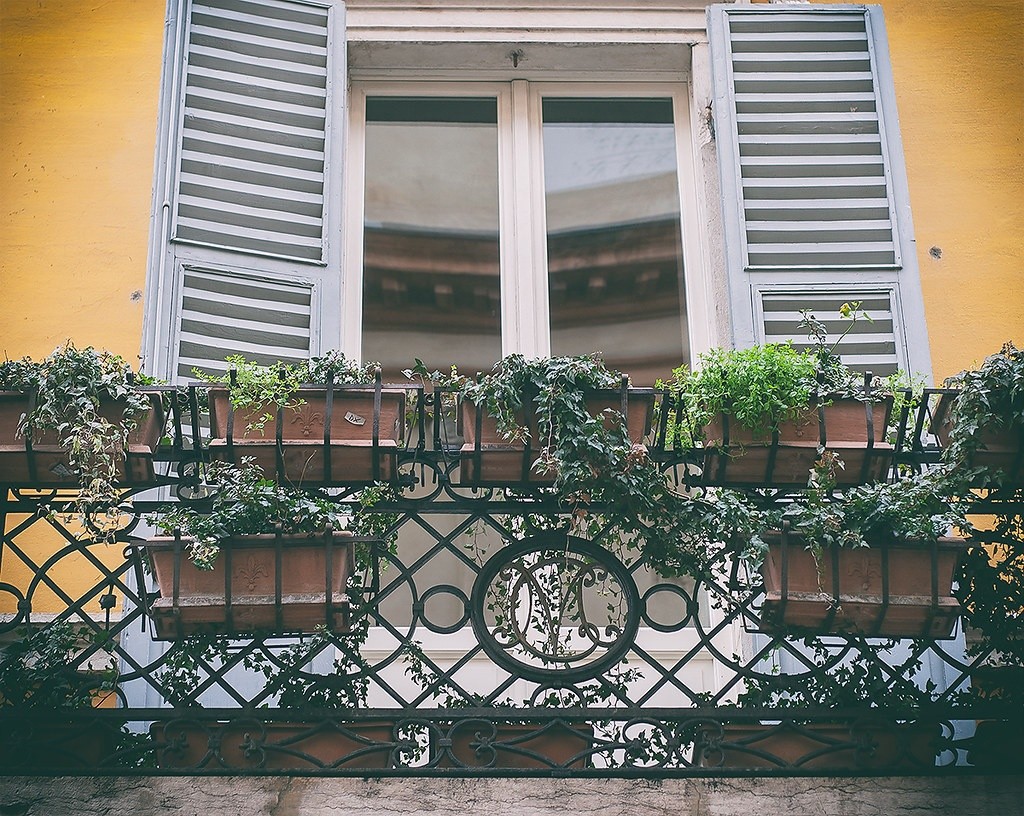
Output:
[0,301,1024,767]
